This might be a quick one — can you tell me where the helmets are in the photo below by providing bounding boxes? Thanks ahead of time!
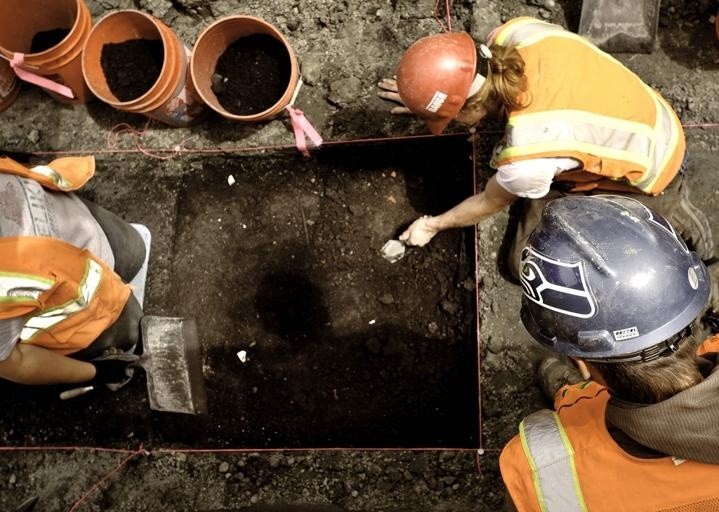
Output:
[519,194,710,359]
[397,30,477,136]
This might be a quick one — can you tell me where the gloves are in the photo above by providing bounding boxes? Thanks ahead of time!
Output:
[88,346,139,392]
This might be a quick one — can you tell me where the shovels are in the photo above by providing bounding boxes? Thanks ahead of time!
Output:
[91,314,210,416]
[379,239,410,264]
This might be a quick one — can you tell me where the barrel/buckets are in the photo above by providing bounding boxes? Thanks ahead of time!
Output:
[81,8,209,127]
[1,0,96,104]
[1,56,22,113]
[188,14,300,122]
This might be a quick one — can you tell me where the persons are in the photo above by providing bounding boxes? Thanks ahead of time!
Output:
[0,154,144,394]
[496,194,719,511]
[376,16,690,247]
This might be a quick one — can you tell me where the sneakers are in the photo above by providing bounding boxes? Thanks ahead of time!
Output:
[538,357,574,398]
[669,185,715,261]
